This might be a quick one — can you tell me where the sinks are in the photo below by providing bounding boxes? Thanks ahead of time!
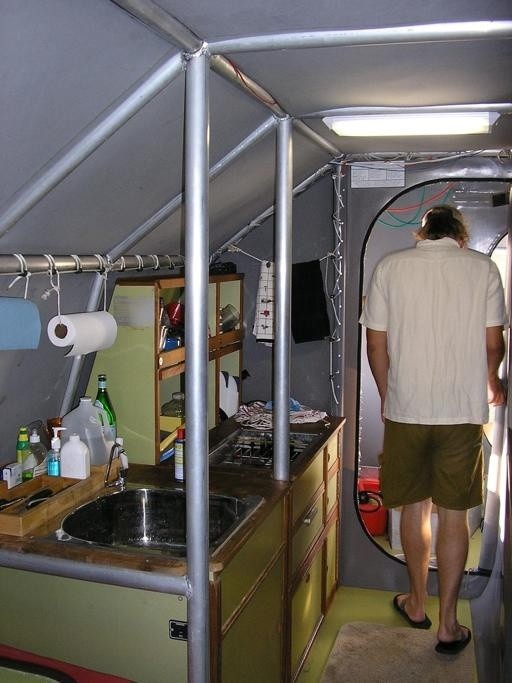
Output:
[60,488,264,560]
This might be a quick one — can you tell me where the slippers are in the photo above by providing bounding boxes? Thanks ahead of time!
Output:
[435,624,472,654]
[393,593,432,629]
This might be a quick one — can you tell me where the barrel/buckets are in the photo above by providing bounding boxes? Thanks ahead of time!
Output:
[61,396,116,466]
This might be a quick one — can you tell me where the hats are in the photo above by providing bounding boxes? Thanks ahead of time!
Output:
[421,205,465,229]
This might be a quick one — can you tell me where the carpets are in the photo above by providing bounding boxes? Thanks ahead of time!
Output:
[318,621,475,683]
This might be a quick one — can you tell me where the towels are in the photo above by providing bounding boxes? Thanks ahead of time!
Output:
[292,260,331,343]
[253,260,274,347]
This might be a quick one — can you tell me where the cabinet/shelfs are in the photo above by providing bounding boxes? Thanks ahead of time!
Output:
[0,273,345,683]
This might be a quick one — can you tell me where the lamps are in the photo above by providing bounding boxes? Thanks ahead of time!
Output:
[323,112,502,135]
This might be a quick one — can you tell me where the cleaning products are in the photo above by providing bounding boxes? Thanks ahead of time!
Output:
[21,428,47,481]
[161,392,185,418]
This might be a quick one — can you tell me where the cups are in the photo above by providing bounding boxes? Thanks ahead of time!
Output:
[219,304,239,330]
[92,374,117,460]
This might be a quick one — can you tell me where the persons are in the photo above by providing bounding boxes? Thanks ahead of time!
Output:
[359,206,507,653]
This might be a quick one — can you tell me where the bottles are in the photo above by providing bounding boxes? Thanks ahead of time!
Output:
[163,391,185,485]
[14,425,47,481]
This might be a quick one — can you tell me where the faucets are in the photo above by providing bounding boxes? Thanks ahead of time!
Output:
[105,444,119,486]
